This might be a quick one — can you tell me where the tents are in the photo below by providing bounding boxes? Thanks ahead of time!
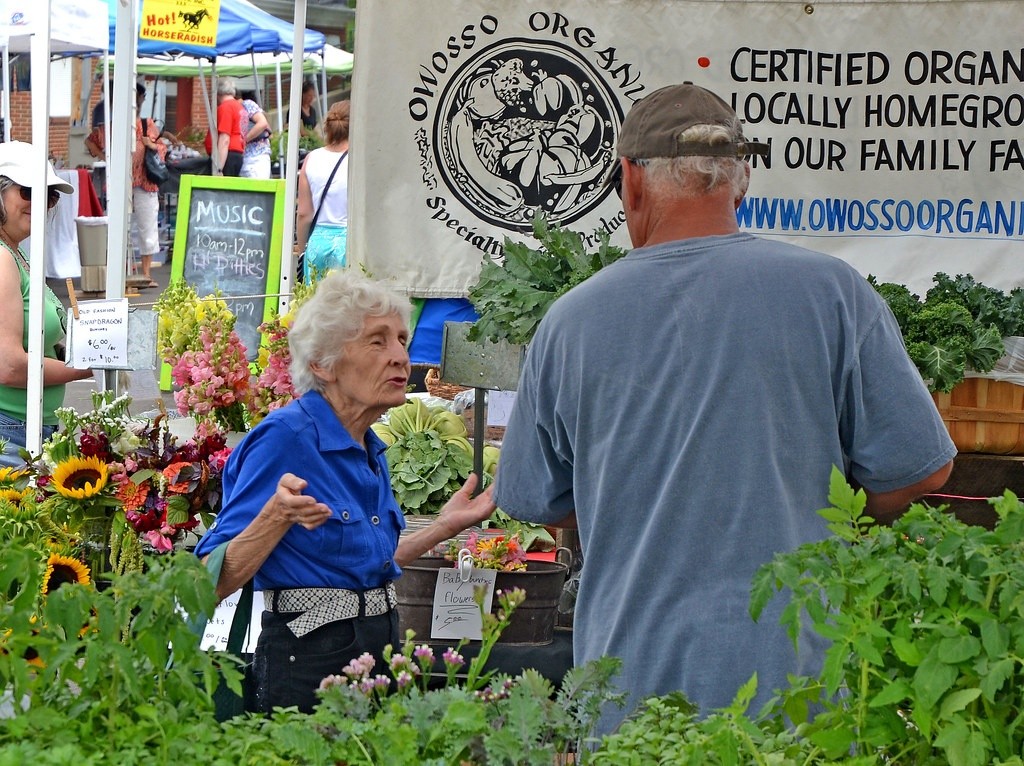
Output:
[0,0,110,201]
[62,0,327,179]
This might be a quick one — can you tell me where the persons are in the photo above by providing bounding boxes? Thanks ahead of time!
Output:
[296,99,350,285]
[84,83,168,287]
[204,78,249,178]
[285,80,317,136]
[491,82,959,753]
[0,140,93,470]
[92,79,114,128]
[234,86,273,179]
[191,269,498,720]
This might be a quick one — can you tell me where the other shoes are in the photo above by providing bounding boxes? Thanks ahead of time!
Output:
[146,277,158,287]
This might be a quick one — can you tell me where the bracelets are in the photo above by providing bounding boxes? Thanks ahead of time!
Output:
[217,169,223,172]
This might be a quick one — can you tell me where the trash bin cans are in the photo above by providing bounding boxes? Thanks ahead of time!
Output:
[73,214,107,294]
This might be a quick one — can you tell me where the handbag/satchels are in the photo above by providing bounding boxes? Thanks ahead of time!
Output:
[141,118,169,187]
[146,540,254,723]
[246,119,271,143]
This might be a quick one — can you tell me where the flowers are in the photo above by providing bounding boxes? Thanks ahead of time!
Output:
[445,528,529,572]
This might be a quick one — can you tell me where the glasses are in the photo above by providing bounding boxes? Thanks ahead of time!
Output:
[611,157,650,200]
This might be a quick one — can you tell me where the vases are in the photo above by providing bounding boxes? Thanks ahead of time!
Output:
[393,556,570,644]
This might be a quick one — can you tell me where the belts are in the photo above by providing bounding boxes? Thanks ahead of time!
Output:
[264,583,398,638]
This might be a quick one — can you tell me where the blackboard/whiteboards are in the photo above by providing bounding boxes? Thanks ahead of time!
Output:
[163,171,287,375]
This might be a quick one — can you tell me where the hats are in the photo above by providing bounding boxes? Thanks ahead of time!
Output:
[0,140,74,194]
[12,182,60,208]
[616,81,770,159]
